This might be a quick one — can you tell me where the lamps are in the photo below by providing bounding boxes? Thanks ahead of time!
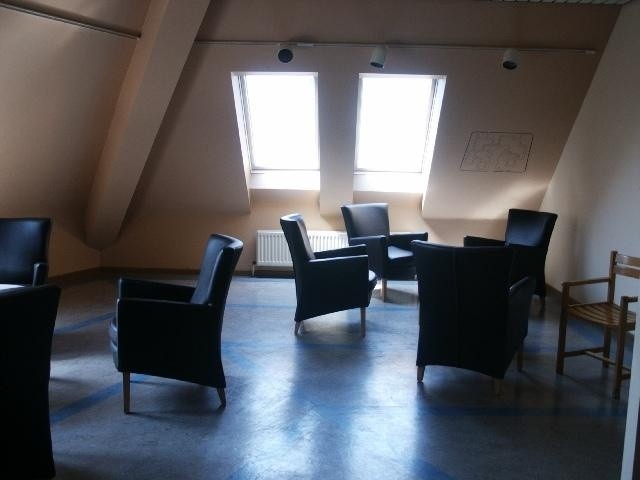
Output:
[499,48,522,70]
[275,42,294,65]
[369,44,388,68]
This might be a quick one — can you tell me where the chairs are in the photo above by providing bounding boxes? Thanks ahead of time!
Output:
[280,213,377,338]
[110,233,244,413]
[556,249,640,401]
[0,286,62,479]
[464,209,557,317]
[1,216,53,285]
[342,203,428,303]
[410,240,533,395]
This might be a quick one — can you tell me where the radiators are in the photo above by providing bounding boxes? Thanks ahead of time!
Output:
[255,230,350,269]
